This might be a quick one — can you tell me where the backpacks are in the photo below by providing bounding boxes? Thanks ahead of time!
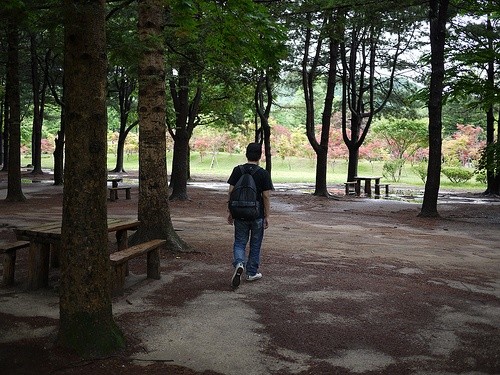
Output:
[226,165,263,221]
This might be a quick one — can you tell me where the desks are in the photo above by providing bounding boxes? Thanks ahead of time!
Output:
[354,176,383,198]
[107,179,123,199]
[13,219,141,293]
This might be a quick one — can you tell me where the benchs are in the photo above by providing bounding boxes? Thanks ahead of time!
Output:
[376,184,390,198]
[109,240,167,297]
[0,240,60,290]
[108,187,131,201]
[344,181,357,195]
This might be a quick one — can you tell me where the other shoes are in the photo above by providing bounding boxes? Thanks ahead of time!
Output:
[245,273,263,281]
[232,262,244,289]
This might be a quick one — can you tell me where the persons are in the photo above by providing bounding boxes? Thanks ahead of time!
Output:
[227,143,273,289]
